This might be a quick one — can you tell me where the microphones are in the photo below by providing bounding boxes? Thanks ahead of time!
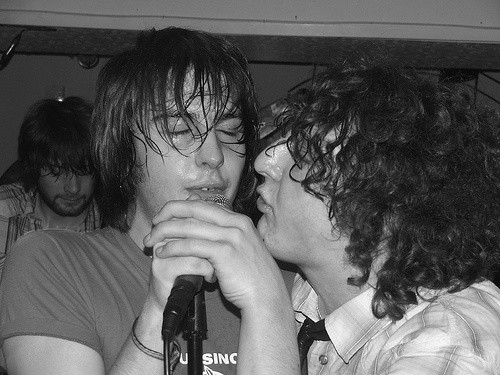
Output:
[161,194,233,339]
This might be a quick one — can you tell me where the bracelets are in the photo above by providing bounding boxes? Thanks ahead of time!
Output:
[128,318,183,369]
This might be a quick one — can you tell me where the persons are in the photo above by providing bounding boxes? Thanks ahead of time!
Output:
[0,25,262,375]
[254,51,499,375]
[0,96,110,278]
